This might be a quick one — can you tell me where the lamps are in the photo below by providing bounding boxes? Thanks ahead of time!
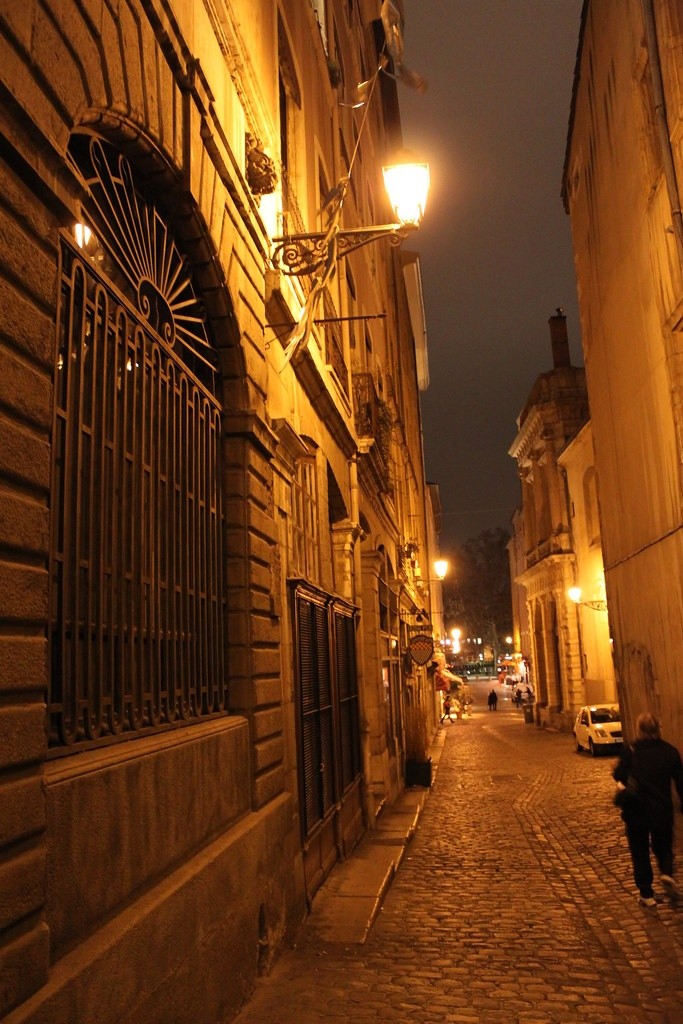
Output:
[268,160,430,275]
[415,559,447,590]
[570,588,607,611]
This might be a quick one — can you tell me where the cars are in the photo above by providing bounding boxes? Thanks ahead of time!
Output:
[573,704,624,755]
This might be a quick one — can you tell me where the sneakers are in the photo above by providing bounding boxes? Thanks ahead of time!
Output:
[660,875,677,894]
[639,897,656,907]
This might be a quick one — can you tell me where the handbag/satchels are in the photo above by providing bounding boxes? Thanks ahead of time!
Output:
[612,776,638,808]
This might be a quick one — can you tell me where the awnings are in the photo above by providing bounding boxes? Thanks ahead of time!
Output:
[435,669,462,691]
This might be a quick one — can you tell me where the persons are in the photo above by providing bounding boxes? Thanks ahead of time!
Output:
[440,696,455,724]
[488,689,497,710]
[516,689,522,707]
[612,712,683,907]
[524,687,531,703]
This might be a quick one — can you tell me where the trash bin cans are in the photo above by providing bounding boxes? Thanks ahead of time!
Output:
[523,704,534,723]
